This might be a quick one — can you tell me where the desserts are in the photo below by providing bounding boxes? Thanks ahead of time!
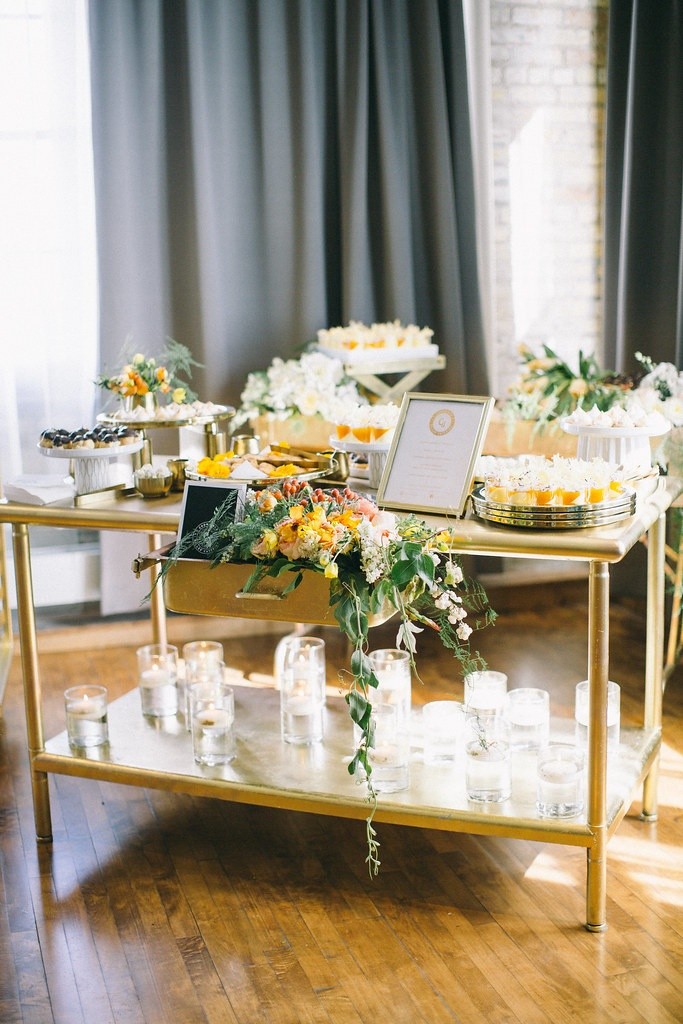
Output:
[482,402,664,506]
[318,318,434,445]
[38,399,226,450]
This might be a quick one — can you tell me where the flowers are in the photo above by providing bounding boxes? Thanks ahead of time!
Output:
[497,342,628,456]
[147,479,496,881]
[89,338,205,409]
[228,353,360,436]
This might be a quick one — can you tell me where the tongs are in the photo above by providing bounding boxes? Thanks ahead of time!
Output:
[257,445,337,467]
[75,463,162,506]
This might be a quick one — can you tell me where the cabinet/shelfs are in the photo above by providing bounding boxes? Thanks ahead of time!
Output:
[0,456,683,934]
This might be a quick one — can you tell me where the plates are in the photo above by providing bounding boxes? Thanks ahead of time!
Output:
[471,487,636,528]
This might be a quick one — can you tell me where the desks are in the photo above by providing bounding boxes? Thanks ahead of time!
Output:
[484,408,683,698]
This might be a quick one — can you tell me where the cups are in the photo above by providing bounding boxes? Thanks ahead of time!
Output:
[367,649,411,720]
[168,459,189,492]
[232,435,258,455]
[423,700,465,755]
[462,715,512,803]
[137,645,179,716]
[190,686,238,766]
[63,685,108,746]
[507,689,550,755]
[537,745,586,819]
[464,669,507,718]
[283,637,325,707]
[281,672,325,745]
[576,682,620,727]
[352,702,395,773]
[183,641,223,687]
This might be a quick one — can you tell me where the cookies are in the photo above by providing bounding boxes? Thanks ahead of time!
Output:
[216,452,317,474]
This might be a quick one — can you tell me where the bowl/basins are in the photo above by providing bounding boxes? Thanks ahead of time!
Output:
[135,473,173,499]
